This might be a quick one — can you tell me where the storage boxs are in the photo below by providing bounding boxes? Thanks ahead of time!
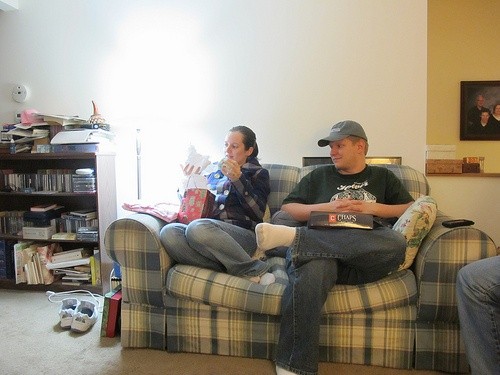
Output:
[30,203,64,226]
[100,284,121,337]
[22,226,56,240]
[31,137,50,153]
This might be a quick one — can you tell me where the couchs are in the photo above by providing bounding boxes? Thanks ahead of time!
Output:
[103,163,496,374]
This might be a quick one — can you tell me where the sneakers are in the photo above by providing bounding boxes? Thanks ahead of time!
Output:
[59,298,81,329]
[71,300,98,333]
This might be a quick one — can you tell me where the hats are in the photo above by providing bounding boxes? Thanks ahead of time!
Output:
[318,120,367,148]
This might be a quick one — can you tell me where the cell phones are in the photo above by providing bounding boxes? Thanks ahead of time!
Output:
[442,220,474,228]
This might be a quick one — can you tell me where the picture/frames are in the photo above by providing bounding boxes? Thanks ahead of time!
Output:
[459,81,500,141]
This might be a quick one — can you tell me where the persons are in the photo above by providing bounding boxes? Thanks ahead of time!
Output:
[254,120,416,375]
[466,94,500,141]
[160,125,276,285]
[455,255,500,375]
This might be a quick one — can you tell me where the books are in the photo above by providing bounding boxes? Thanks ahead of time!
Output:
[0,203,99,240]
[0,167,96,194]
[13,241,101,286]
[43,116,86,127]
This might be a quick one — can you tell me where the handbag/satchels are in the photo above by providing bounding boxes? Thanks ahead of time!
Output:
[177,175,216,225]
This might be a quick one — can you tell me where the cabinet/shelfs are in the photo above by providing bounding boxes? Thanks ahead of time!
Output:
[0,152,117,296]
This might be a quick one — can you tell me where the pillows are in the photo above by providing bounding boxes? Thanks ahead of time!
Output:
[393,194,437,271]
